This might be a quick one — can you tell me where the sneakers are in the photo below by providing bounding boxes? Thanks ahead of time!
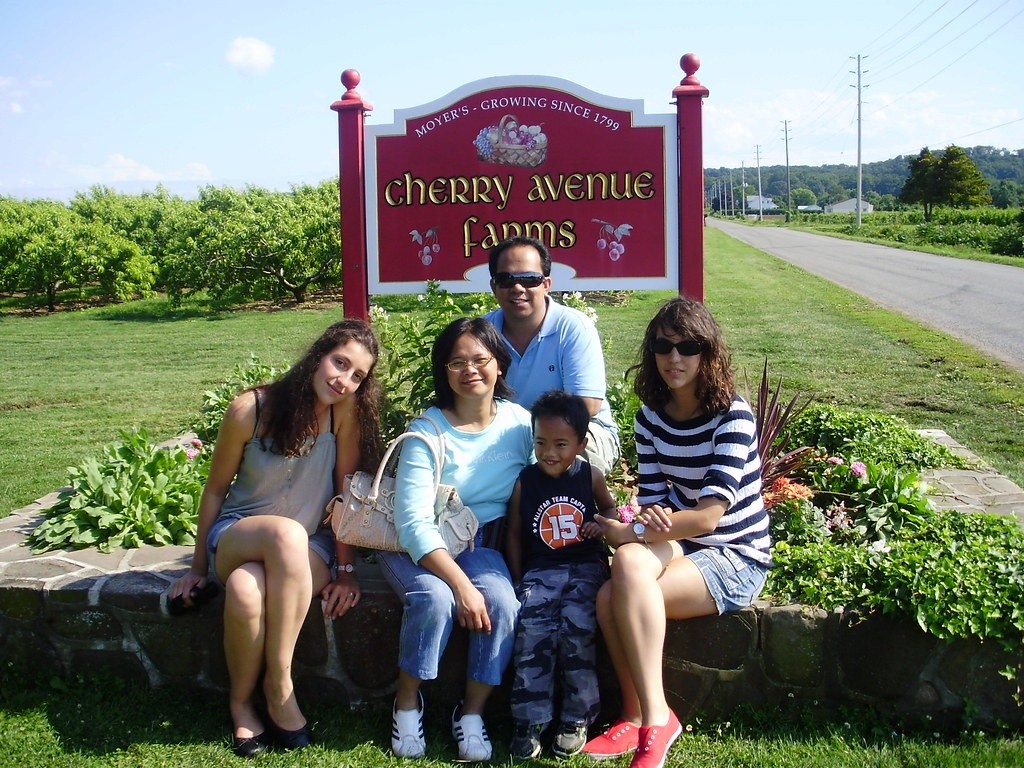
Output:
[551,719,589,757]
[391,688,426,758]
[451,700,493,762]
[509,721,550,759]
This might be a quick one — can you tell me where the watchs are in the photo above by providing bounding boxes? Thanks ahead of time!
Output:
[336,563,356,573]
[632,521,646,543]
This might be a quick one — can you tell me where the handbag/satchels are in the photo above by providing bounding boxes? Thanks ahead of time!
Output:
[323,414,479,560]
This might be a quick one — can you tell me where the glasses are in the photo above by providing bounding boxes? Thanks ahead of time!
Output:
[648,337,704,357]
[491,271,546,289]
[444,355,496,372]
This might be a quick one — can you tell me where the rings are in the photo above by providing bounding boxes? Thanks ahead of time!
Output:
[348,592,355,597]
[601,534,608,544]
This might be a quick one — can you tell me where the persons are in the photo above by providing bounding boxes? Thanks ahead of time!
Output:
[507,390,616,760]
[580,298,772,768]
[481,236,621,478]
[169,319,379,758]
[356,317,535,761]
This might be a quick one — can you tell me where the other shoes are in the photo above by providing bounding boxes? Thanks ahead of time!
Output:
[581,719,639,760]
[629,705,683,768]
[261,672,312,750]
[229,699,268,757]
